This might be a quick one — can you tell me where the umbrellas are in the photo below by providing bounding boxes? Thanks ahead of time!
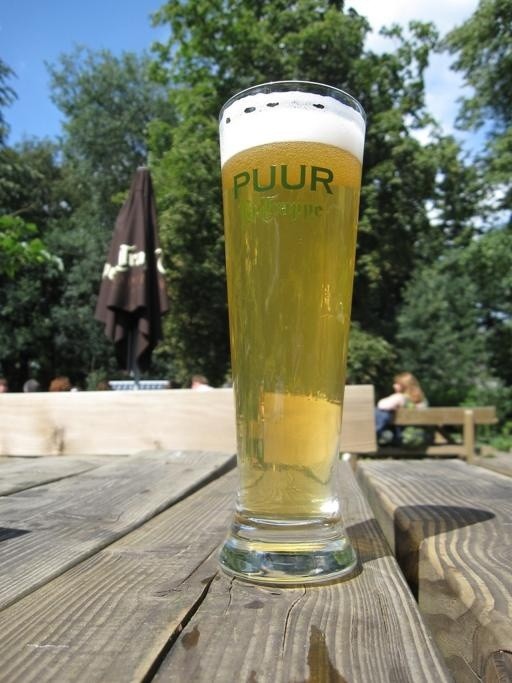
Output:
[93,160,171,373]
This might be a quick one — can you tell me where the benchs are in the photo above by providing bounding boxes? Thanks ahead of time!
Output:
[377,404,500,456]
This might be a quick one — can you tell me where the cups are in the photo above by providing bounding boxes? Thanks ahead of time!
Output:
[218,81,367,586]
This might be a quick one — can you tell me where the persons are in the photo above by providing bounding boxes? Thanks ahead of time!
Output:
[376,373,430,448]
[191,374,210,388]
[96,378,113,390]
[47,376,72,391]
[22,378,41,392]
[0,377,9,392]
